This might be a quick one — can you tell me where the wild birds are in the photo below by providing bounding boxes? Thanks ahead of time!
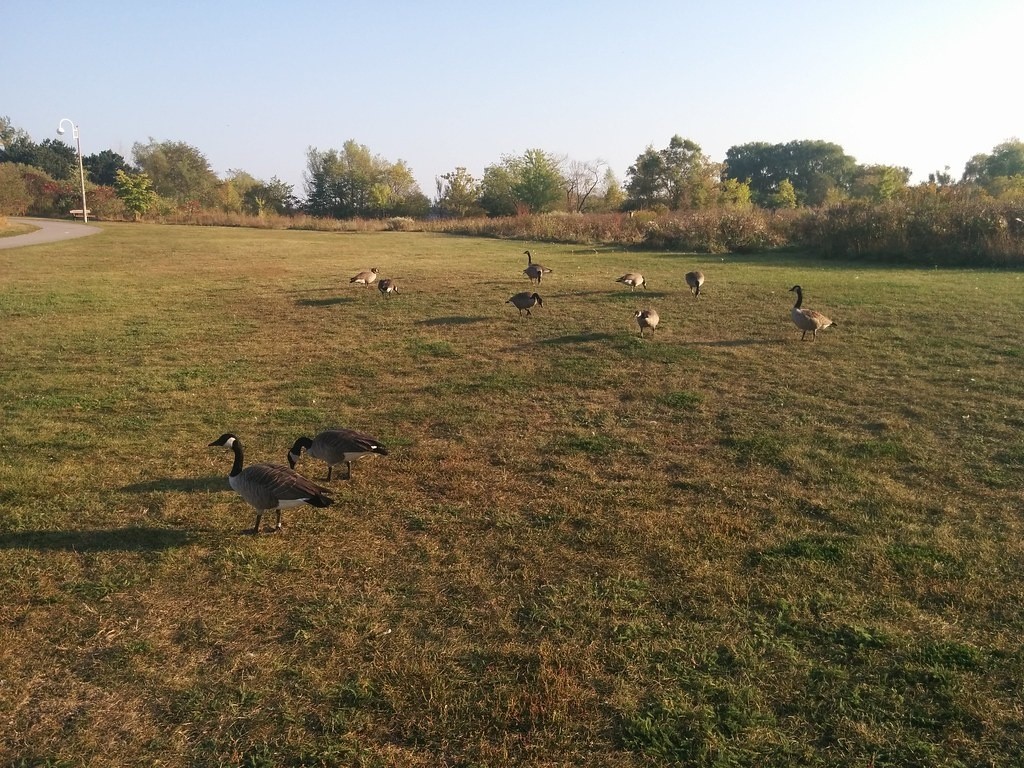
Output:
[504,250,554,316]
[615,270,705,340]
[349,267,401,298]
[207,427,391,537]
[788,284,838,343]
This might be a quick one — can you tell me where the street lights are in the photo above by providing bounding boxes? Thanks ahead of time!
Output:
[56,119,89,224]
